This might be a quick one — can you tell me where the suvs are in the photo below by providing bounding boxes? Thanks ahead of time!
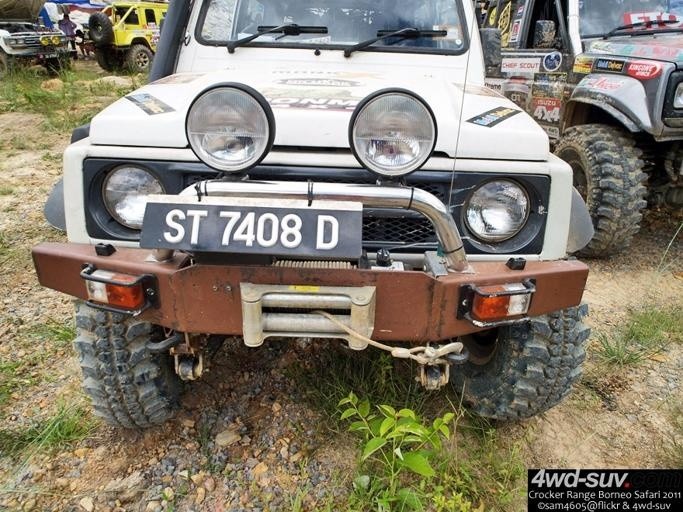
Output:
[23,0,594,437]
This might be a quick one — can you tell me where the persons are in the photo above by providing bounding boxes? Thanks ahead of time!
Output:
[59,14,77,60]
[75,29,97,61]
